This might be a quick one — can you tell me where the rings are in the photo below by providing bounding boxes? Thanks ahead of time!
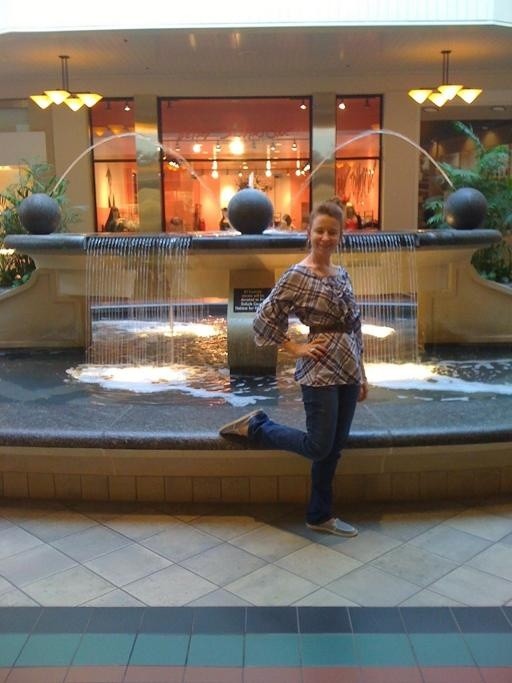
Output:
[310,344,317,351]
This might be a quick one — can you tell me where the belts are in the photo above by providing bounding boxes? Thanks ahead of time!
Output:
[310,323,355,332]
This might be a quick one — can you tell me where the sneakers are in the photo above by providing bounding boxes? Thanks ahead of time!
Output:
[219,408,264,437]
[305,517,358,537]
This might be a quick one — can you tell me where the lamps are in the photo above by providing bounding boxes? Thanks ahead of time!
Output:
[407,50,482,108]
[299,100,307,110]
[29,56,103,111]
[339,98,346,110]
[174,134,298,152]
[123,100,130,111]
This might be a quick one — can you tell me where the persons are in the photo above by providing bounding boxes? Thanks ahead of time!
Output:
[219,212,290,229]
[345,200,361,229]
[218,196,369,538]
[104,207,128,234]
